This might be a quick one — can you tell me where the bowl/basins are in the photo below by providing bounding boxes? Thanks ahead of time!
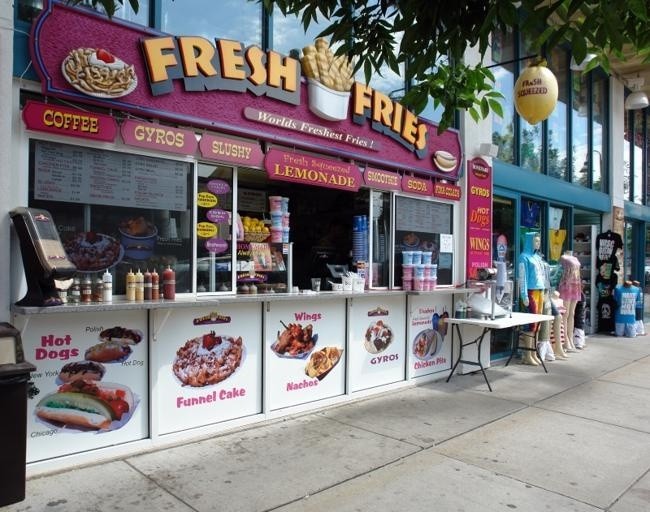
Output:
[119,226,158,259]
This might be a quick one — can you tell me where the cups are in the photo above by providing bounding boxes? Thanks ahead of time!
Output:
[402,251,437,292]
[342,277,353,291]
[352,214,368,267]
[269,196,291,243]
[433,312,450,338]
[311,277,321,291]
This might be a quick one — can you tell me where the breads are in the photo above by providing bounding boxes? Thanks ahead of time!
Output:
[301,38,353,92]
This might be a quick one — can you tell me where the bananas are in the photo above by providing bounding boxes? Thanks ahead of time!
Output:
[436,155,456,168]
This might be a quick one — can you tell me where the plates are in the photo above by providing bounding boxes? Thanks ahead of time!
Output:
[413,329,442,362]
[66,233,124,273]
[434,150,457,173]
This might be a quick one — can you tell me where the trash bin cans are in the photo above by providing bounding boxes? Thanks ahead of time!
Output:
[0,321,36,500]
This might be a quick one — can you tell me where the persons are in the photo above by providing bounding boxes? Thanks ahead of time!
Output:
[614,280,636,338]
[320,216,345,247]
[516,231,587,366]
[633,280,645,335]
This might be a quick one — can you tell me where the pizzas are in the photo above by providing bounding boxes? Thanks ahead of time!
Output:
[173,331,242,387]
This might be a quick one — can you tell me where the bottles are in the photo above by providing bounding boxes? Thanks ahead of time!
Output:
[455,298,472,318]
[126,265,176,301]
[242,281,258,293]
[72,268,113,302]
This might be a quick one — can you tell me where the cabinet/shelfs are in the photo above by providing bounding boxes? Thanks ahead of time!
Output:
[573,223,598,335]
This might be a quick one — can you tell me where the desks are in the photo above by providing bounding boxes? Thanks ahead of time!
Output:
[443,311,556,394]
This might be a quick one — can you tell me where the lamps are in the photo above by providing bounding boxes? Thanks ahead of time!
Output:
[482,53,560,125]
[624,73,649,110]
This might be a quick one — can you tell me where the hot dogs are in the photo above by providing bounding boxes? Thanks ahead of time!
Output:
[85,341,130,361]
[100,327,141,343]
[35,391,114,428]
[60,361,105,380]
